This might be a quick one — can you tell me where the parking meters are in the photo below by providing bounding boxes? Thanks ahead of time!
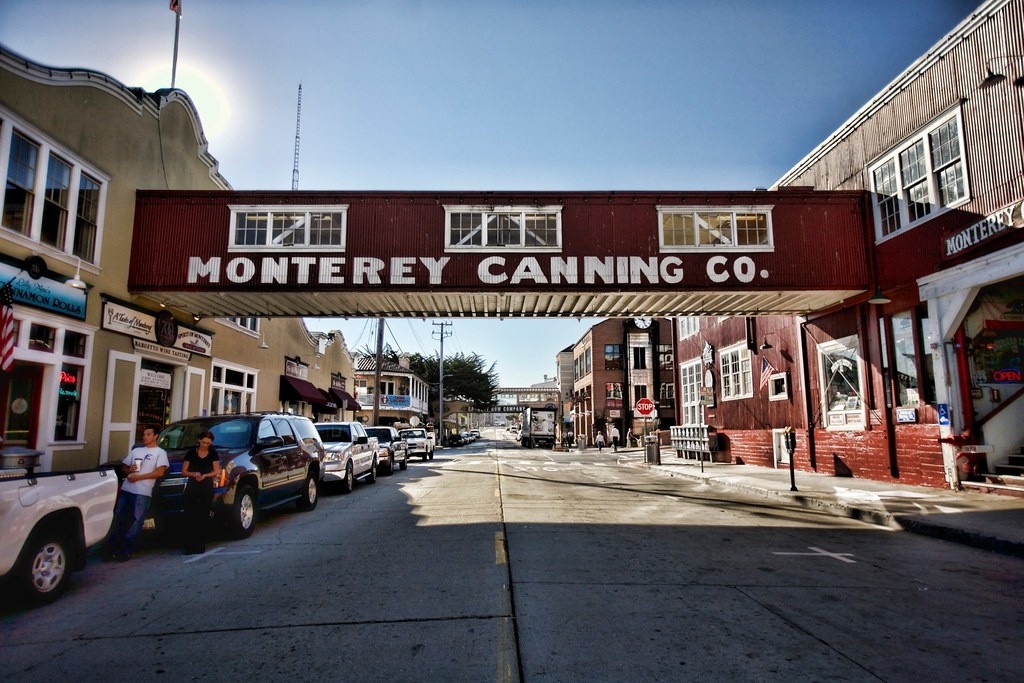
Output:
[784,426,797,491]
[655,429,661,464]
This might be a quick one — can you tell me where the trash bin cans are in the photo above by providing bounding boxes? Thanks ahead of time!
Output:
[578,434,586,451]
[645,436,660,465]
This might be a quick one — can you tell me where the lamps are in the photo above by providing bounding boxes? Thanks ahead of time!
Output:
[259,331,268,349]
[760,332,774,349]
[194,312,202,321]
[160,298,169,307]
[32,250,86,289]
[302,353,320,370]
[979,55,1023,88]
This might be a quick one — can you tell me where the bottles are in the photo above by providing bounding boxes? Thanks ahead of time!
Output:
[382,395,388,404]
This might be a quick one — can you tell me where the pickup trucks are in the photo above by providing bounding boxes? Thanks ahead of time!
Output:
[0,466,119,602]
[313,421,380,493]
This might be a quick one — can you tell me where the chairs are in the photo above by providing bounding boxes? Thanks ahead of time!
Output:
[867,280,896,304]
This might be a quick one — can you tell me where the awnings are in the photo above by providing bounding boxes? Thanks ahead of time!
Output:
[280,374,361,413]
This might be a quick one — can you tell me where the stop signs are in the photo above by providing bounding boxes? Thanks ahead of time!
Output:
[636,398,654,416]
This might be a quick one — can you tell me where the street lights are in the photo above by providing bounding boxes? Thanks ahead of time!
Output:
[439,374,453,446]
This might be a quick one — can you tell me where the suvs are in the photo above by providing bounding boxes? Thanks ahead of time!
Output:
[96,411,326,540]
[470,429,480,438]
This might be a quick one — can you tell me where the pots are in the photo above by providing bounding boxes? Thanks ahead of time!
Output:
[0,446,45,468]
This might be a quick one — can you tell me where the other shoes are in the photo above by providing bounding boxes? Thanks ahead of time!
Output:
[183,547,204,555]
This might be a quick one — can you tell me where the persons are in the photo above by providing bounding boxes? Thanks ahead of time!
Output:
[611,425,620,452]
[541,419,548,435]
[595,431,606,454]
[98,426,169,561]
[562,429,573,448]
[626,428,634,448]
[181,431,220,555]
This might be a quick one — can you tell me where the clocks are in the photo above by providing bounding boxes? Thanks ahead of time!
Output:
[634,318,652,329]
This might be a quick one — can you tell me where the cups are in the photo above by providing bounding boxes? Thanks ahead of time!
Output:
[134,458,143,471]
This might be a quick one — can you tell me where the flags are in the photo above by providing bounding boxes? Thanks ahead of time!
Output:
[0,281,15,374]
[760,357,774,390]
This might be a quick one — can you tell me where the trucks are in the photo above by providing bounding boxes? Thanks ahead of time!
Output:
[397,428,435,461]
[520,407,558,449]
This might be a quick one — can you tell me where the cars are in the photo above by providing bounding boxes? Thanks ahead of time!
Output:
[506,427,511,431]
[510,426,522,442]
[485,421,505,427]
[363,426,409,476]
[448,432,476,447]
